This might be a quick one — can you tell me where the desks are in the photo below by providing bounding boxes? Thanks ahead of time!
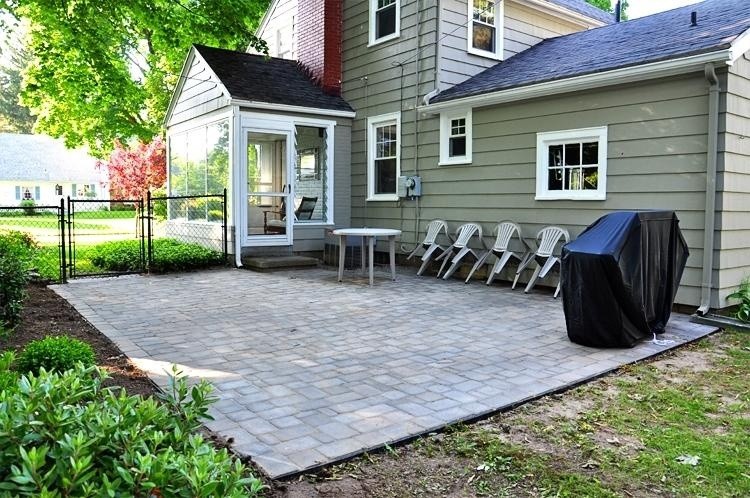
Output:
[332,227,402,285]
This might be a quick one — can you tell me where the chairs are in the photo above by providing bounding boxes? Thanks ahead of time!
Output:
[405,218,571,299]
[263,196,319,234]
